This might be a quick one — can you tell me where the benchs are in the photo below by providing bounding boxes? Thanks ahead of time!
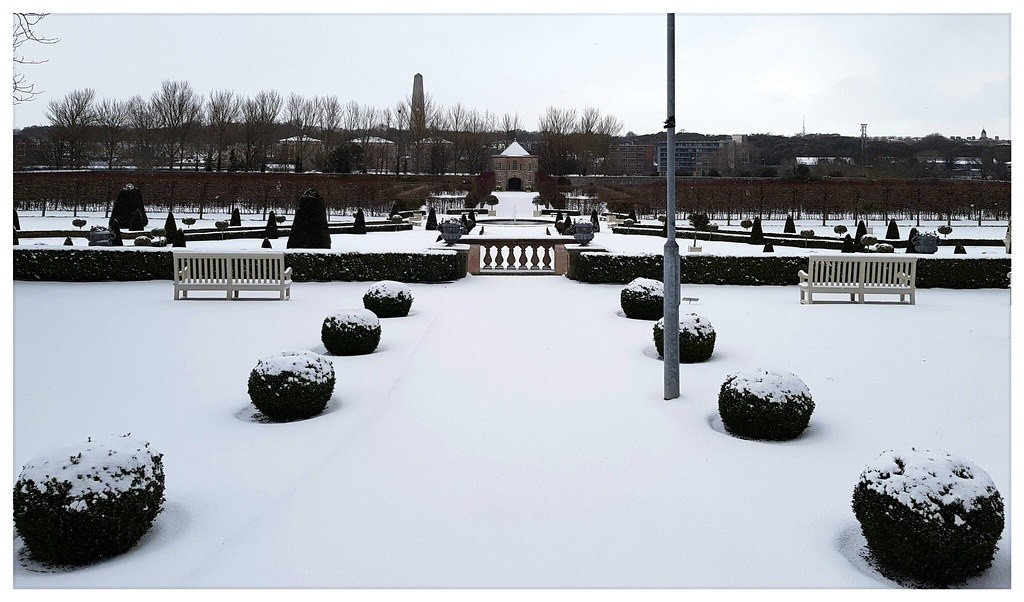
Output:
[798,256,916,306]
[172,250,293,301]
